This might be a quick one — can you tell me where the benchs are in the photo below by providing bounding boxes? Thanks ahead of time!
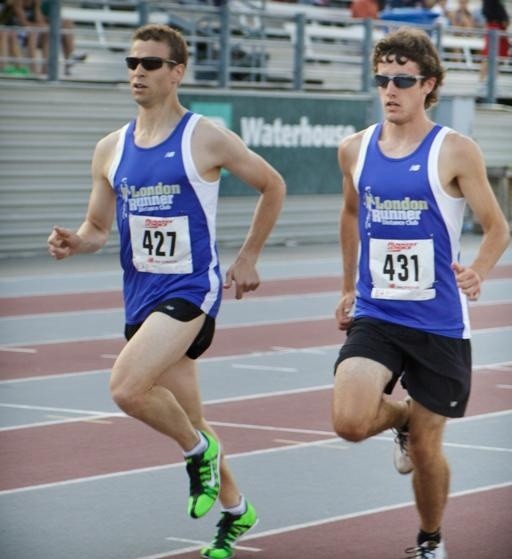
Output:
[0,3,200,89]
[230,0,511,105]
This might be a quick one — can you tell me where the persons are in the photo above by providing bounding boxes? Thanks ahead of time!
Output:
[47,23,286,559]
[332,26,510,559]
[0,0,88,75]
[351,0,512,86]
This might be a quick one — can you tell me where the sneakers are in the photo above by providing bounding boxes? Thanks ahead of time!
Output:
[418,528,446,559]
[199,497,258,558]
[184,429,221,519]
[393,397,416,474]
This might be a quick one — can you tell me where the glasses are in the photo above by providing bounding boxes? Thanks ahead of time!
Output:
[374,73,426,89]
[125,56,178,71]
